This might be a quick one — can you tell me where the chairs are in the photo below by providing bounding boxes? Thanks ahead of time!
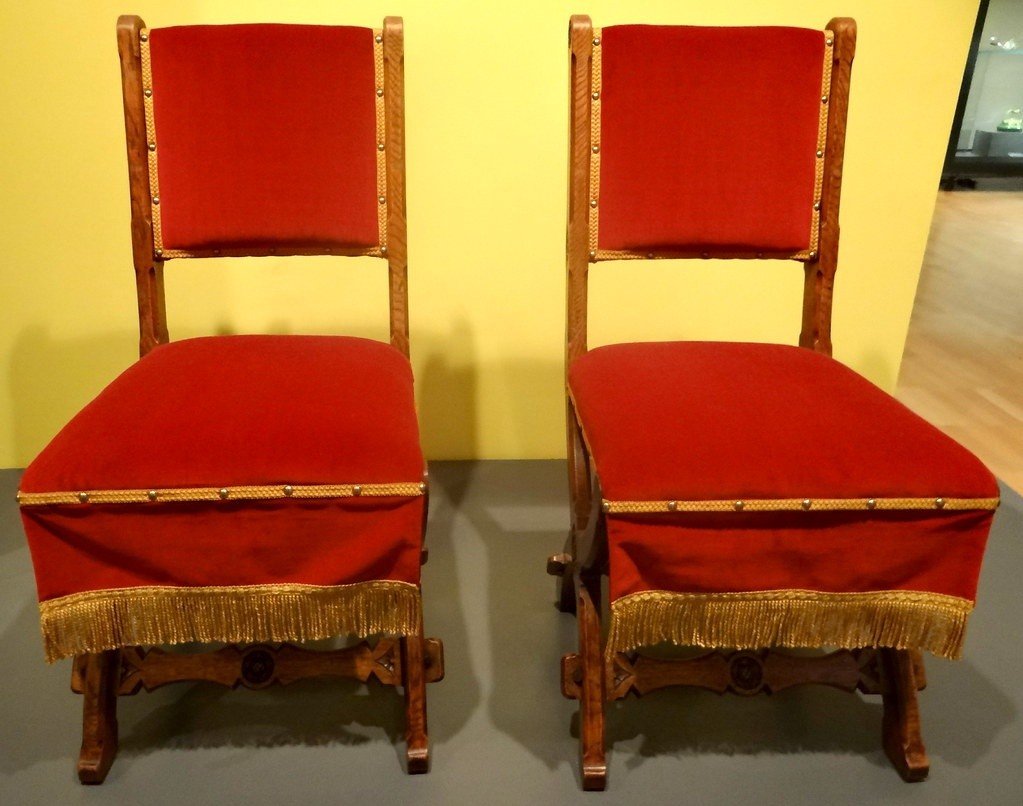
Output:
[13,13,444,776]
[546,13,1005,790]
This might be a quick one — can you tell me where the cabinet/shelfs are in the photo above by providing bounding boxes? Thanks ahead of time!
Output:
[941,0,1023,193]
